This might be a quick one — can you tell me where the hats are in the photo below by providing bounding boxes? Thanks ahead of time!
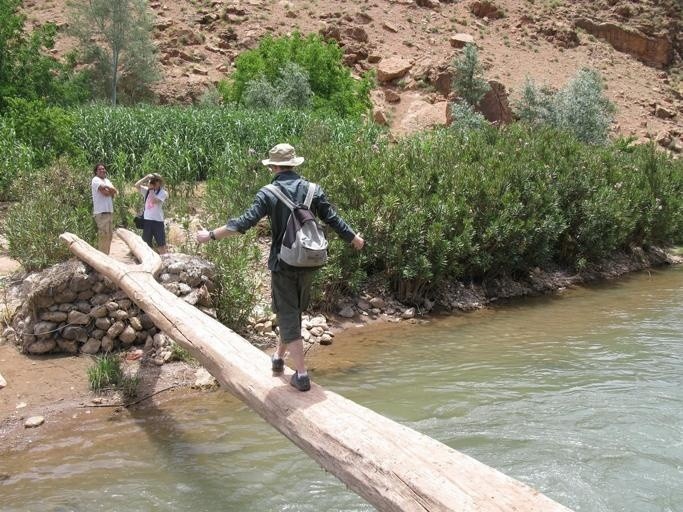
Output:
[262,144,304,167]
[148,173,165,186]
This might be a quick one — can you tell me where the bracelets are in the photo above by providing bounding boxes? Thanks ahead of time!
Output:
[210,230,216,241]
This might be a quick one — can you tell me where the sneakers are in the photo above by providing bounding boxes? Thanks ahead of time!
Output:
[290,370,310,390]
[271,354,284,372]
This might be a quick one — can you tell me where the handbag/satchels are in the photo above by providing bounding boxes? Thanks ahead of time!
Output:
[134,216,144,229]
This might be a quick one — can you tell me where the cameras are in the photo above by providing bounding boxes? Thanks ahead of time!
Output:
[150,179,155,184]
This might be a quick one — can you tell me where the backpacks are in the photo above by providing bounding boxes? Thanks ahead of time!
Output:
[263,183,328,271]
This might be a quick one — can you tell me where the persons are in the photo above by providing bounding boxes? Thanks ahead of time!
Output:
[91,163,119,254]
[200,143,365,391]
[134,172,170,254]
